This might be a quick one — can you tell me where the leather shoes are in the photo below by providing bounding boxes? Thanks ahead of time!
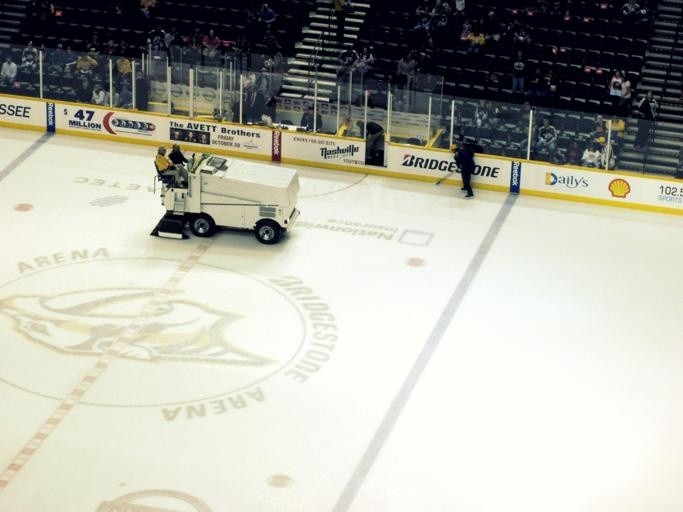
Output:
[462,187,468,190]
[465,193,473,197]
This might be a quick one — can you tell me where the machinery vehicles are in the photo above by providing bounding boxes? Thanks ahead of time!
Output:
[150,151,302,246]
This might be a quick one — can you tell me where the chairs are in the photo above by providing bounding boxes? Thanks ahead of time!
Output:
[0,1,322,123]
[336,0,660,164]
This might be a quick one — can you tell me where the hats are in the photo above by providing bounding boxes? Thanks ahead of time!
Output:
[598,137,605,143]
[451,144,456,149]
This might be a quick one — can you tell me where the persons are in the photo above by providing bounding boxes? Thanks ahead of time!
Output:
[167,143,189,184]
[1,0,658,171]
[153,147,185,188]
[449,143,477,198]
